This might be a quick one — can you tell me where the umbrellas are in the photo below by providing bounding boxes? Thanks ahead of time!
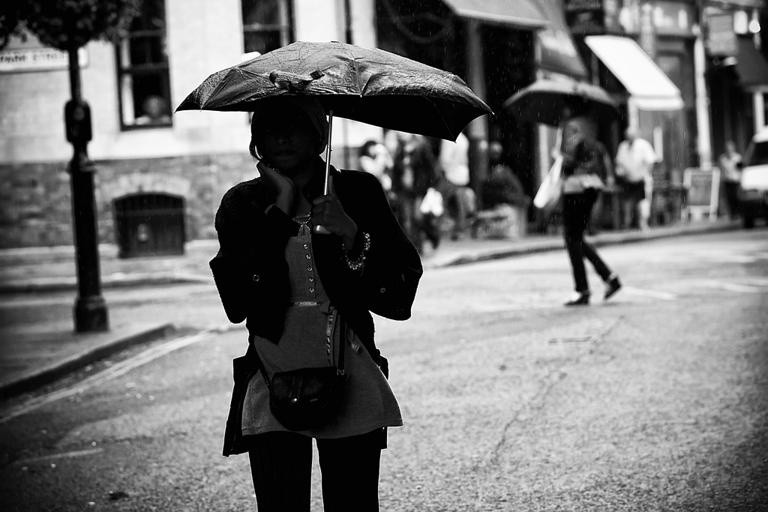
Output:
[504,77,623,134]
[173,41,496,234]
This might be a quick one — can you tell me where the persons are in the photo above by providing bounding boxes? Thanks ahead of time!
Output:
[209,97,423,512]
[135,95,172,125]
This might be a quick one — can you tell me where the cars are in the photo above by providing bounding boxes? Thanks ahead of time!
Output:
[736,127,768,231]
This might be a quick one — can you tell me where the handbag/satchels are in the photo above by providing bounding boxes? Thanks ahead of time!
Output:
[534,156,565,218]
[269,366,349,433]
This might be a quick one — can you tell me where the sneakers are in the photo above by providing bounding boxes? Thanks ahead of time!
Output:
[566,294,589,305]
[604,278,621,298]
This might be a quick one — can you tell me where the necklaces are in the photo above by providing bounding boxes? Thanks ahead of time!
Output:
[291,211,312,225]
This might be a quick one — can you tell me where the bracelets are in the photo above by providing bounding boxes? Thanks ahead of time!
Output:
[341,231,371,270]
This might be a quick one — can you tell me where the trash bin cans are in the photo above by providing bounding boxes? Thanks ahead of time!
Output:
[114,192,186,258]
